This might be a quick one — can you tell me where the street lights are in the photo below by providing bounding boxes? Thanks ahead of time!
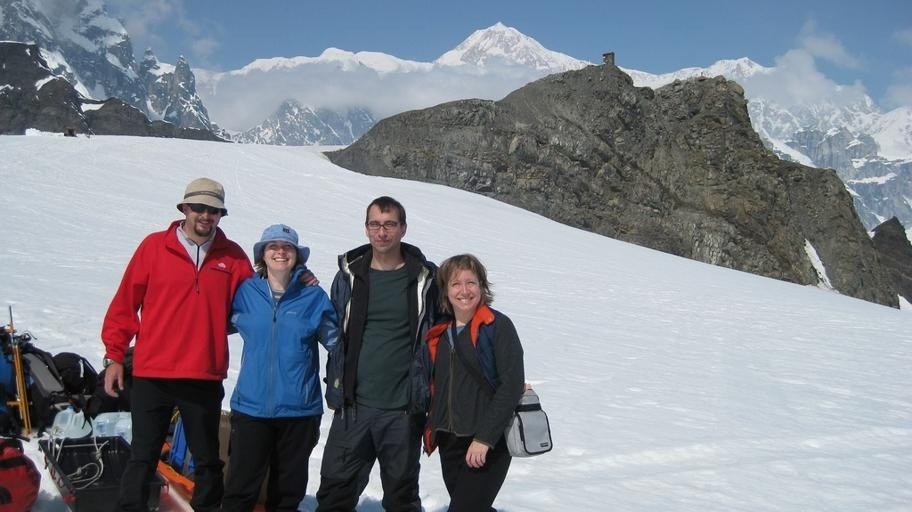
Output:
[504,383,553,457]
[0,437,39,512]
[54,353,98,394]
[17,341,69,425]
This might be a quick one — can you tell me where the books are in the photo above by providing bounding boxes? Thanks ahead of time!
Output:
[38,435,168,512]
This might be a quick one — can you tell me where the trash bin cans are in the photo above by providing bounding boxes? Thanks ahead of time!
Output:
[104,359,115,367]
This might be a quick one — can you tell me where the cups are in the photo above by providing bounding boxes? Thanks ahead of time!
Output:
[190,204,219,214]
[368,222,398,231]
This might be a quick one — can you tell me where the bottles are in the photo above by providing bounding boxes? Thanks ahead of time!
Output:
[177,178,228,216]
[254,225,309,264]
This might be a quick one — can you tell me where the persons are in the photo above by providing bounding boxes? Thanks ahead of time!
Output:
[317,197,442,512]
[102,178,252,512]
[229,221,343,512]
[418,253,525,511]
